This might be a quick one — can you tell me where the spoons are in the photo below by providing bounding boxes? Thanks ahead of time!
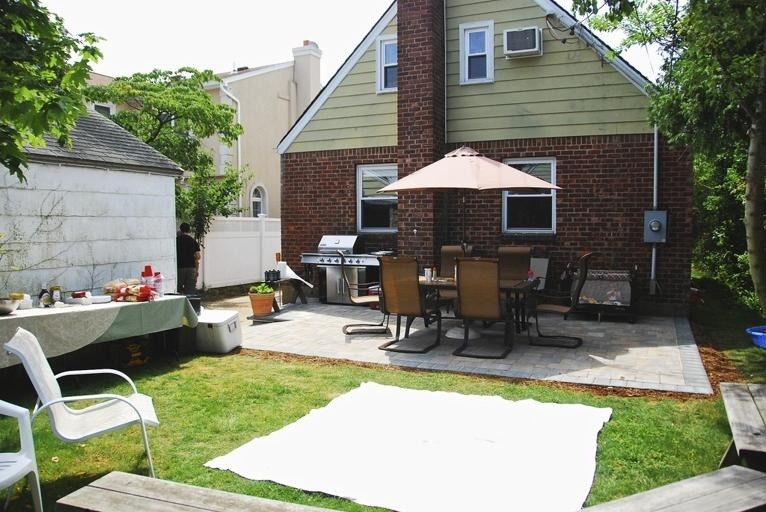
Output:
[512,279,526,287]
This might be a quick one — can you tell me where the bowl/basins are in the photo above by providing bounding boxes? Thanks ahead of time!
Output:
[747,325,766,349]
[0,299,21,316]
[20,300,33,310]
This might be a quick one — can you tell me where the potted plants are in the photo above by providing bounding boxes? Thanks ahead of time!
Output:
[249,283,274,315]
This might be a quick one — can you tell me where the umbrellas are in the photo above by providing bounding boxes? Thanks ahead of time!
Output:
[376,146,565,258]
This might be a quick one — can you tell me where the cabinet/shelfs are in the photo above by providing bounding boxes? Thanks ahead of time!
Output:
[316,264,367,305]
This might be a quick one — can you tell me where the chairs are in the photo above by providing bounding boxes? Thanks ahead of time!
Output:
[5,326,159,479]
[377,257,441,353]
[336,249,389,334]
[496,244,532,333]
[453,256,513,359]
[526,249,602,349]
[0,400,44,512]
[438,245,472,316]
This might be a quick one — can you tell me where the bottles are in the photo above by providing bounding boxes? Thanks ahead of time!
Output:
[424,266,437,282]
[526,268,534,286]
[263,269,280,282]
[37,282,51,307]
[48,284,61,303]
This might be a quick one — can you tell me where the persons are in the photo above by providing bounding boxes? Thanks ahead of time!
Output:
[176,222,201,296]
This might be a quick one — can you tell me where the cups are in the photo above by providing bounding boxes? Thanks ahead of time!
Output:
[141,265,162,294]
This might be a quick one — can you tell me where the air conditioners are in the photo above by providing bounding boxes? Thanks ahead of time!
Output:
[502,26,542,57]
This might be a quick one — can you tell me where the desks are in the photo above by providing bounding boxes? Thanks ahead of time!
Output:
[0,296,199,367]
[264,278,307,312]
[418,274,541,334]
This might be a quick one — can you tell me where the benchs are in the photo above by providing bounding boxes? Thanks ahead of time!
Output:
[718,382,766,469]
[579,464,765,511]
[56,470,351,512]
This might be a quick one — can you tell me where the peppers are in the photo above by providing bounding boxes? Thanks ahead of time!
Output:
[249,282,273,294]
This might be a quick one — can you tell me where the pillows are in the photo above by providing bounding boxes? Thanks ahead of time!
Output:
[179,307,242,354]
